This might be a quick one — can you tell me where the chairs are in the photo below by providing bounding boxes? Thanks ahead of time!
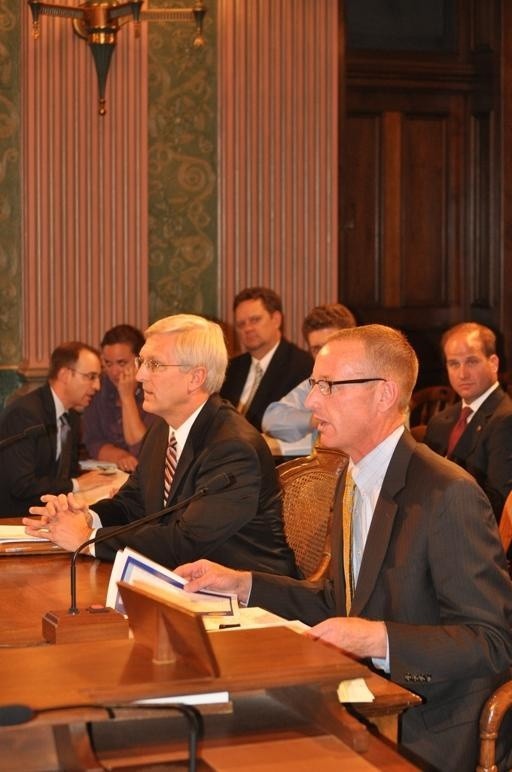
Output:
[409,386,455,443]
[274,452,349,583]
[475,680,511,772]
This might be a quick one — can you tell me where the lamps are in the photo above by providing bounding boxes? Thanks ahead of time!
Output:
[26,0,207,117]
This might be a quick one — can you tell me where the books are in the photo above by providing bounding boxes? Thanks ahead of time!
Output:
[0,542,76,556]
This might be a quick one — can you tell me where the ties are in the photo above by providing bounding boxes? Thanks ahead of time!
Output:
[448,406,474,453]
[59,413,72,482]
[242,364,262,417]
[164,432,176,505]
[341,472,356,619]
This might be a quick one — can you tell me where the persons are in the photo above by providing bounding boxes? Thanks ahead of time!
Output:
[21,314,307,580]
[0,342,120,517]
[171,323,512,772]
[82,325,156,473]
[422,321,512,526]
[220,287,314,434]
[260,304,358,462]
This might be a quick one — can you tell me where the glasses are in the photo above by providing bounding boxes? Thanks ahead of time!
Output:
[71,366,101,382]
[135,357,190,373]
[307,379,388,396]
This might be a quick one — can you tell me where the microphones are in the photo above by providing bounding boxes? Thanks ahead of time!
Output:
[42,471,235,643]
[0,703,203,771]
